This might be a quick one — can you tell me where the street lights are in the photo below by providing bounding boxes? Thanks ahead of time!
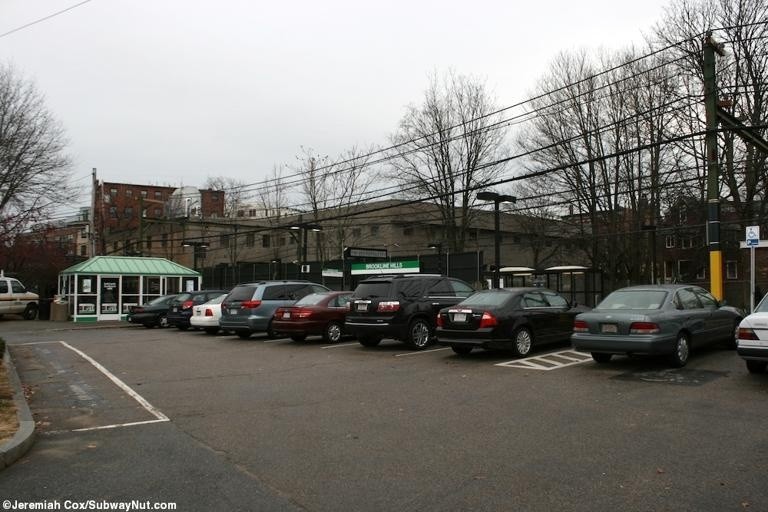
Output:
[287,223,323,262]
[476,191,516,289]
[180,240,211,271]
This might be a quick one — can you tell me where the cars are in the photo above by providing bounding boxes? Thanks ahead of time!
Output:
[568,280,749,371]
[732,289,767,373]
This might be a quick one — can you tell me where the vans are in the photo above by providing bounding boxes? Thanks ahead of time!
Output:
[0,276,40,320]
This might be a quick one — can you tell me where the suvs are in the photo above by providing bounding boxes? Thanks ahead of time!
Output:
[341,271,479,349]
[218,278,336,339]
